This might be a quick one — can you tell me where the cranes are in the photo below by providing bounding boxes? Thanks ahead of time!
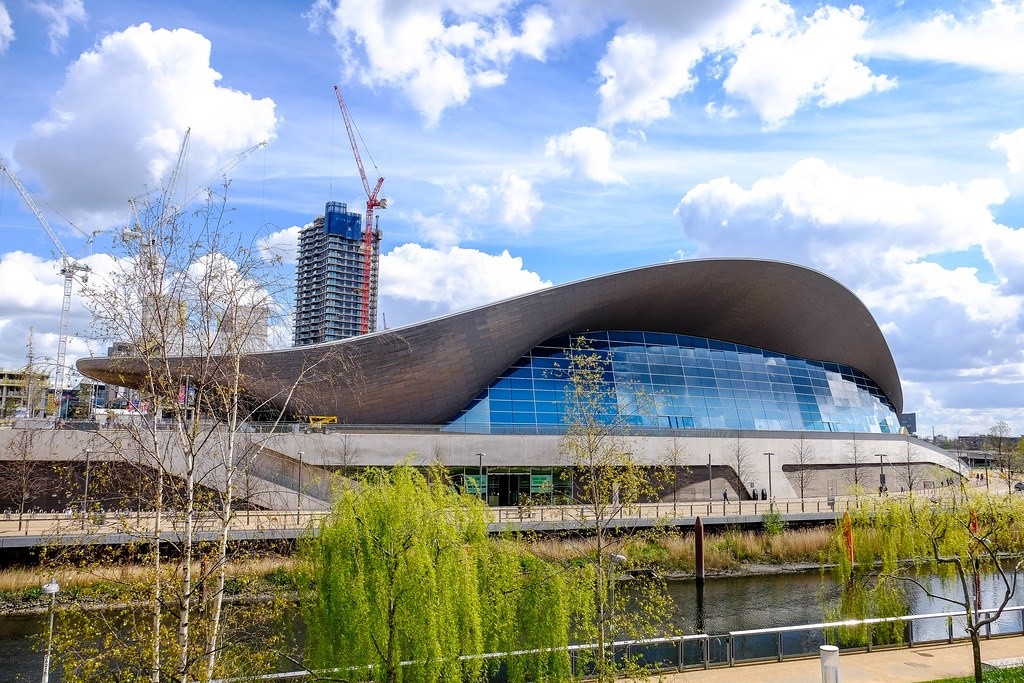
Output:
[120,126,270,340]
[331,83,386,336]
[0,163,94,420]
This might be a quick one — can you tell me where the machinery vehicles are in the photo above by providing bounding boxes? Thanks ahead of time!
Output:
[302,413,337,435]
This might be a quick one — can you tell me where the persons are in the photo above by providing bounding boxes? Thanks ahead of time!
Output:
[900,485,905,494]
[723,489,730,505]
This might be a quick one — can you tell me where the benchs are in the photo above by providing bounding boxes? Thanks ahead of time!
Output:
[981,657,1024,671]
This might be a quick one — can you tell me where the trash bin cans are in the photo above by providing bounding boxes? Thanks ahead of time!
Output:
[489,495,499,506]
[924,480,934,489]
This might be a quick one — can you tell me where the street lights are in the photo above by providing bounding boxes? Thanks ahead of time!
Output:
[294,451,305,525]
[475,452,486,499]
[973,536,992,638]
[81,447,93,530]
[623,451,636,515]
[763,452,776,511]
[608,550,628,666]
[875,453,888,493]
[41,577,61,683]
[978,453,995,487]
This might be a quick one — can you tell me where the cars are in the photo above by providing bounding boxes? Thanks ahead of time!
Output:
[1014,482,1024,492]
